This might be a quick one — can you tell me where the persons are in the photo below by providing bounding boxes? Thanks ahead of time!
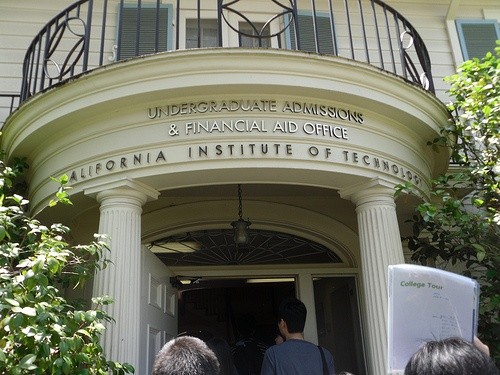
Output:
[151,335,221,375]
[404,334,500,375]
[260,298,334,375]
[229,307,285,375]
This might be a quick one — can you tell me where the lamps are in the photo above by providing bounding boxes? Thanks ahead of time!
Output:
[231,184,251,247]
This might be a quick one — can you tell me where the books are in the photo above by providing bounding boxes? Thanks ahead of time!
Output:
[386,264,481,373]
